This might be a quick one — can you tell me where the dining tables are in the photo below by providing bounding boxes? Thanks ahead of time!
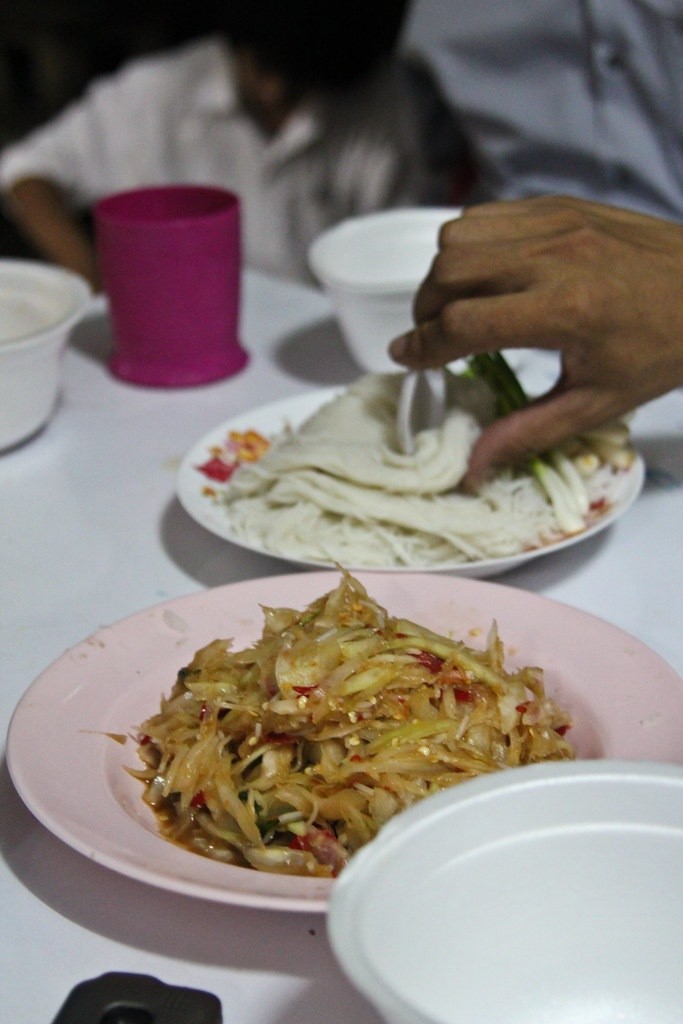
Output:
[0,292,683,1024]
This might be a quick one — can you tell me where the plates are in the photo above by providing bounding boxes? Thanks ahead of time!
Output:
[6,571,683,914]
[175,384,646,579]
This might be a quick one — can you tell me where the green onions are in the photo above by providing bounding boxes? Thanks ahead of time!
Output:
[465,346,588,537]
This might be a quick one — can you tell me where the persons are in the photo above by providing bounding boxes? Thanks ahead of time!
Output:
[400,0,683,225]
[0,0,444,293]
[388,192,683,489]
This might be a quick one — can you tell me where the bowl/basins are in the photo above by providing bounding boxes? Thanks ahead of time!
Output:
[0,254,94,455]
[308,205,463,374]
[324,757,683,1024]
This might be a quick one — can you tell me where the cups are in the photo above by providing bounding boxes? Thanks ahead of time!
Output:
[95,180,248,387]
